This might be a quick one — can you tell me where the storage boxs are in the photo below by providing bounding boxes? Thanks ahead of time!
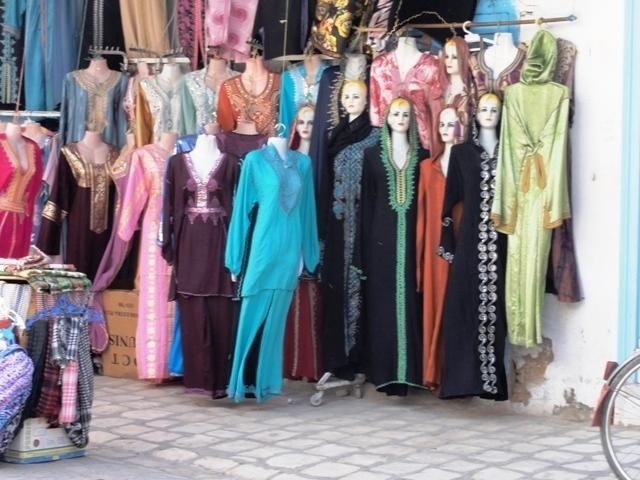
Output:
[90,283,146,381]
